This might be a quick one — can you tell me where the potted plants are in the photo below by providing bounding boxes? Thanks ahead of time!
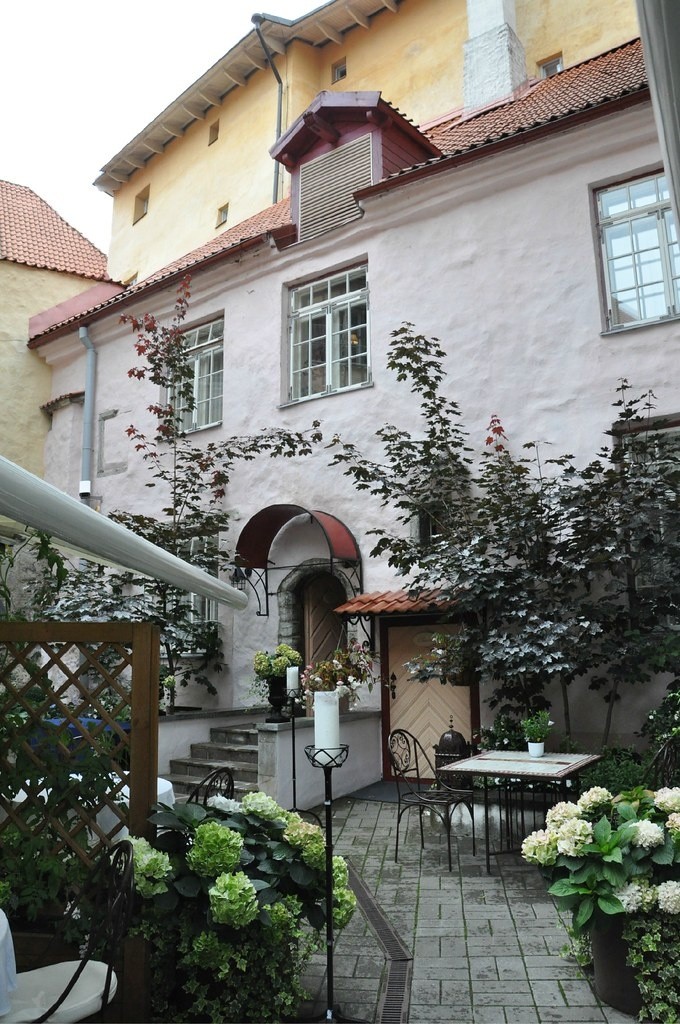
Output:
[521,711,554,758]
[404,623,482,686]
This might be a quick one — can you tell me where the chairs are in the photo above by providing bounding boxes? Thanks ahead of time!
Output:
[0,839,135,1024]
[186,765,235,809]
[388,728,477,872]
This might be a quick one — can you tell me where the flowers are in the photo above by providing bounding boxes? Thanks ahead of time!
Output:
[522,786,680,930]
[254,643,303,679]
[300,637,378,696]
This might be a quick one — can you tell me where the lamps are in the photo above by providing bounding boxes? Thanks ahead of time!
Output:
[282,666,302,699]
[229,550,270,616]
[303,691,349,770]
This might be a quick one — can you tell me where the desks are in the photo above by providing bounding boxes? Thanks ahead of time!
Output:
[436,750,605,875]
[31,717,130,771]
[0,770,176,860]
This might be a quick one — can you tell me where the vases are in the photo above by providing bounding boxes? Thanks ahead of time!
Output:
[306,693,350,717]
[590,906,680,1019]
[266,677,291,723]
